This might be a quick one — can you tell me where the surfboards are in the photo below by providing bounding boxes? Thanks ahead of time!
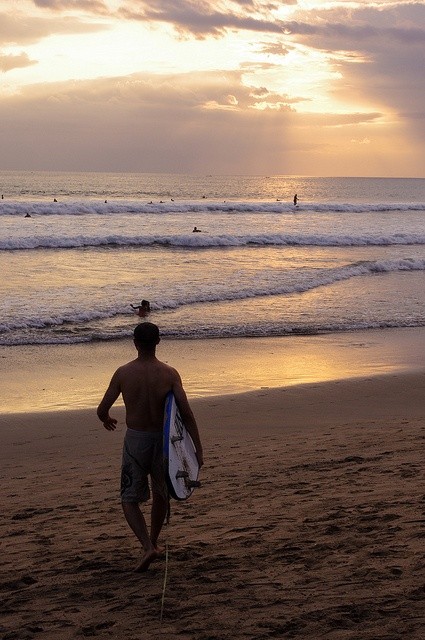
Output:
[163,391,201,500]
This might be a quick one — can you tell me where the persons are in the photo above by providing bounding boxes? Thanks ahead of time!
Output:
[130,300,150,317]
[193,227,201,232]
[293,194,299,205]
[97,322,204,573]
